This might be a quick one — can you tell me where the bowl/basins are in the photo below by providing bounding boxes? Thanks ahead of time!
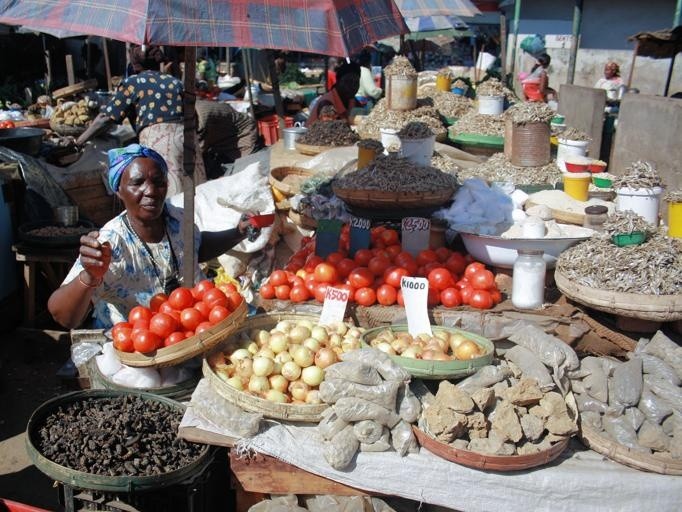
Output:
[249,213,275,228]
[588,164,609,173]
[564,162,589,173]
[591,174,613,188]
[0,127,47,158]
[551,116,565,123]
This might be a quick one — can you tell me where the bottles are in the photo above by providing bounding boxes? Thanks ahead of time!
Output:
[511,247,548,310]
[584,205,610,235]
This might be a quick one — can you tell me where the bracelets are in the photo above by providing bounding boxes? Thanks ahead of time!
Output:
[72,138,80,148]
[79,268,105,290]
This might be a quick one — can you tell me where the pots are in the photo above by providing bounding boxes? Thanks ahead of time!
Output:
[282,127,307,150]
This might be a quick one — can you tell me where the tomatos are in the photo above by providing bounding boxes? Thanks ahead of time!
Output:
[111,279,243,355]
[260,225,503,309]
[0,120,14,128]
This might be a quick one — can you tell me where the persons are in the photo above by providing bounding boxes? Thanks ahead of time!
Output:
[267,55,307,87]
[196,56,217,89]
[594,61,625,172]
[479,24,500,68]
[47,143,210,330]
[305,62,361,128]
[324,57,337,93]
[194,99,260,159]
[453,23,477,67]
[127,44,148,75]
[148,48,174,75]
[354,50,383,109]
[521,53,556,103]
[70,44,207,195]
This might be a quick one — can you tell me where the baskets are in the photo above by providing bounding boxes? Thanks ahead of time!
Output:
[359,323,495,380]
[52,78,98,100]
[24,388,212,494]
[294,141,326,156]
[49,120,87,137]
[332,185,455,210]
[14,117,51,128]
[268,165,314,197]
[553,266,682,322]
[201,311,363,424]
[410,423,570,473]
[112,294,249,370]
[576,422,682,476]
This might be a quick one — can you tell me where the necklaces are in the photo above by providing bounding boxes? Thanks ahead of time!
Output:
[126,212,180,297]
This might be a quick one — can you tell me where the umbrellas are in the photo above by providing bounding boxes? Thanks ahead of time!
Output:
[402,17,471,31]
[0,0,484,287]
[394,0,482,16]
[376,29,476,68]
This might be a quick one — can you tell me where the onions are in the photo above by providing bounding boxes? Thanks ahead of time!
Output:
[371,329,481,361]
[208,319,362,405]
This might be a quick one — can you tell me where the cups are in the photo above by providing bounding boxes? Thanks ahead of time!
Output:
[564,172,592,202]
